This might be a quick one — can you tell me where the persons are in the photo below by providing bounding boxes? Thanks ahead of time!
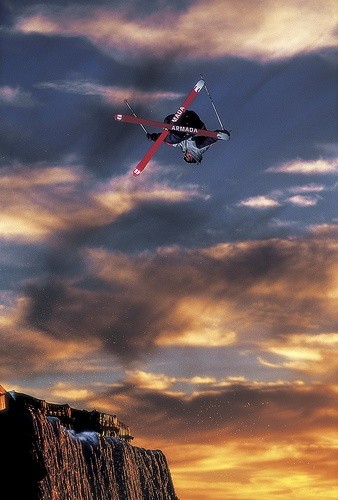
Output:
[145,110,232,164]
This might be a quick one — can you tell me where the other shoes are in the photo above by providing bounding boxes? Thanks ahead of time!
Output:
[150,132,162,141]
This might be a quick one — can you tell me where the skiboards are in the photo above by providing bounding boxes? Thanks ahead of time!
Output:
[112,81,231,178]
